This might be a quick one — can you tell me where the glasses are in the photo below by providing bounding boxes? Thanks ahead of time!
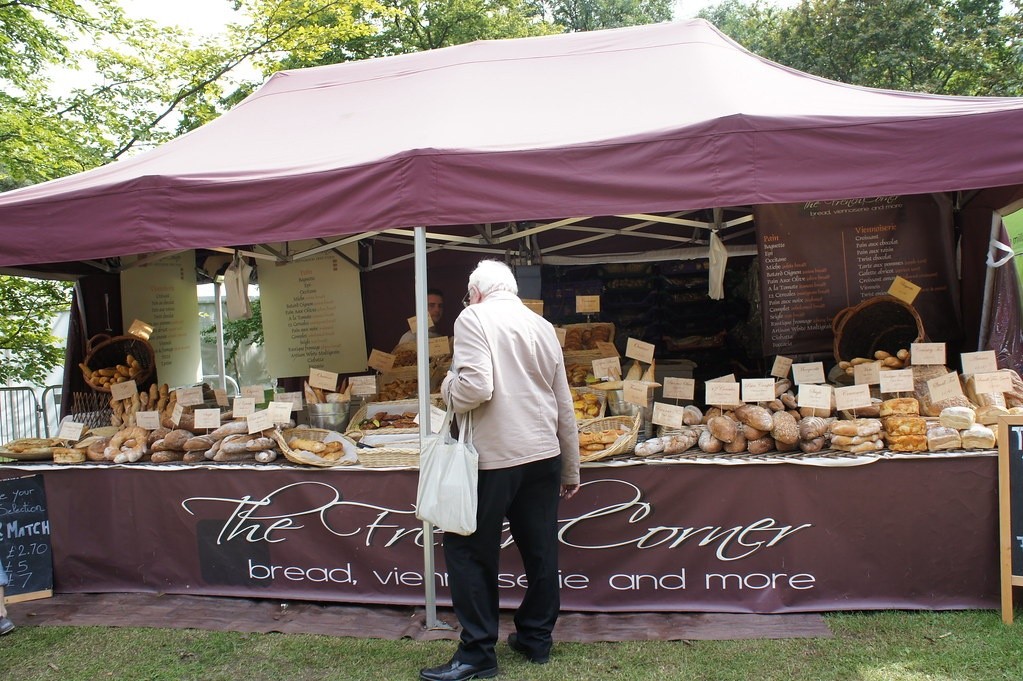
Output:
[461,286,474,307]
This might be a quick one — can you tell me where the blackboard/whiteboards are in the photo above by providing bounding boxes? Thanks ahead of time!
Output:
[996,414,1023,588]
[0,474,54,605]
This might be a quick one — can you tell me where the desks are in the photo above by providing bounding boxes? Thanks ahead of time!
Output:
[0,449,1000,614]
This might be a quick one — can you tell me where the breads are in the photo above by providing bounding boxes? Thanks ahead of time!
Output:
[564,326,1023,451]
[5,352,344,464]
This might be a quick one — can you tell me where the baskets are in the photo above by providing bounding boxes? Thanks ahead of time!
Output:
[560,322,615,354]
[354,435,420,467]
[568,386,607,425]
[656,423,709,438]
[359,365,435,408]
[345,400,446,440]
[437,335,454,364]
[564,355,602,369]
[391,340,436,367]
[277,427,358,466]
[835,372,895,399]
[83,333,155,391]
[430,364,451,395]
[832,295,925,367]
[578,411,641,462]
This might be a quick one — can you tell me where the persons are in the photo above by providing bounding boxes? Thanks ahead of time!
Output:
[419,260,581,681]
[397,289,446,346]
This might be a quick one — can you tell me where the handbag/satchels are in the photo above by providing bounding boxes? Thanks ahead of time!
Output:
[414,394,479,535]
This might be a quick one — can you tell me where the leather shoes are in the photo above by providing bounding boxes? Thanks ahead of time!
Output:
[508,632,549,663]
[419,658,498,681]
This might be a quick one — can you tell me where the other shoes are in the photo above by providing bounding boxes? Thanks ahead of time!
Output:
[0,615,15,634]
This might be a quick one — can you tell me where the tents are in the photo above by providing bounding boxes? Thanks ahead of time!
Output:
[0,19,1023,631]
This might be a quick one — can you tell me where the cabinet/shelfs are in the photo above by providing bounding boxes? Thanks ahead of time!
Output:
[599,269,728,337]
